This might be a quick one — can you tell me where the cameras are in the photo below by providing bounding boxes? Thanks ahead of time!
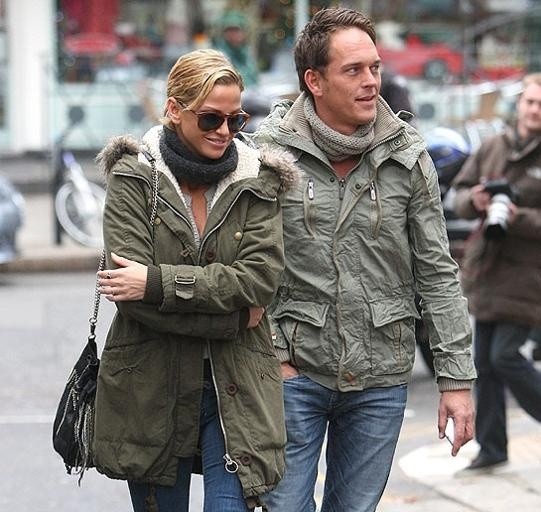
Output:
[481,179,519,239]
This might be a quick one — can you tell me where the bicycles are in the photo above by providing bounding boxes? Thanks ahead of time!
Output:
[34,104,106,247]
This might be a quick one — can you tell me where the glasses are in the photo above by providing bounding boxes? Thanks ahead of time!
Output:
[163,101,249,133]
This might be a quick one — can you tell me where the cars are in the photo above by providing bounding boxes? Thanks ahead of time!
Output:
[372,23,462,80]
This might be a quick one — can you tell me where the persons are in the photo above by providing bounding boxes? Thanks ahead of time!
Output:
[454,73,541,471]
[96,50,303,512]
[214,10,259,87]
[255,8,478,512]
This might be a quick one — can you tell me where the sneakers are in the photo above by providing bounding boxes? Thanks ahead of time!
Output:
[454,457,511,479]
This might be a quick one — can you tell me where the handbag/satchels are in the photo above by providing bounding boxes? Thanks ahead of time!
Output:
[51,335,100,476]
